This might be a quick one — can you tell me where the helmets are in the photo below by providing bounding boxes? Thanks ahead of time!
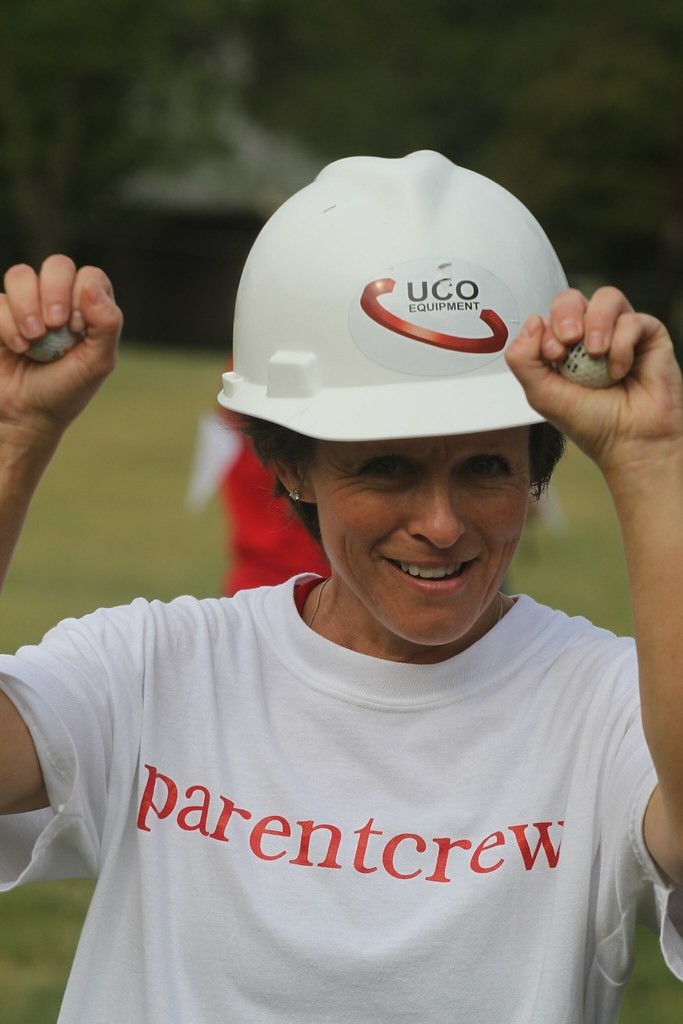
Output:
[217,148,571,443]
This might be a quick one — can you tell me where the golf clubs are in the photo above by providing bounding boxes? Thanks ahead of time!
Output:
[16,315,82,364]
[556,340,634,390]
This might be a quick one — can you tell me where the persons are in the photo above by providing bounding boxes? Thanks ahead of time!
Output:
[0,151,682,1024]
[184,356,332,598]
